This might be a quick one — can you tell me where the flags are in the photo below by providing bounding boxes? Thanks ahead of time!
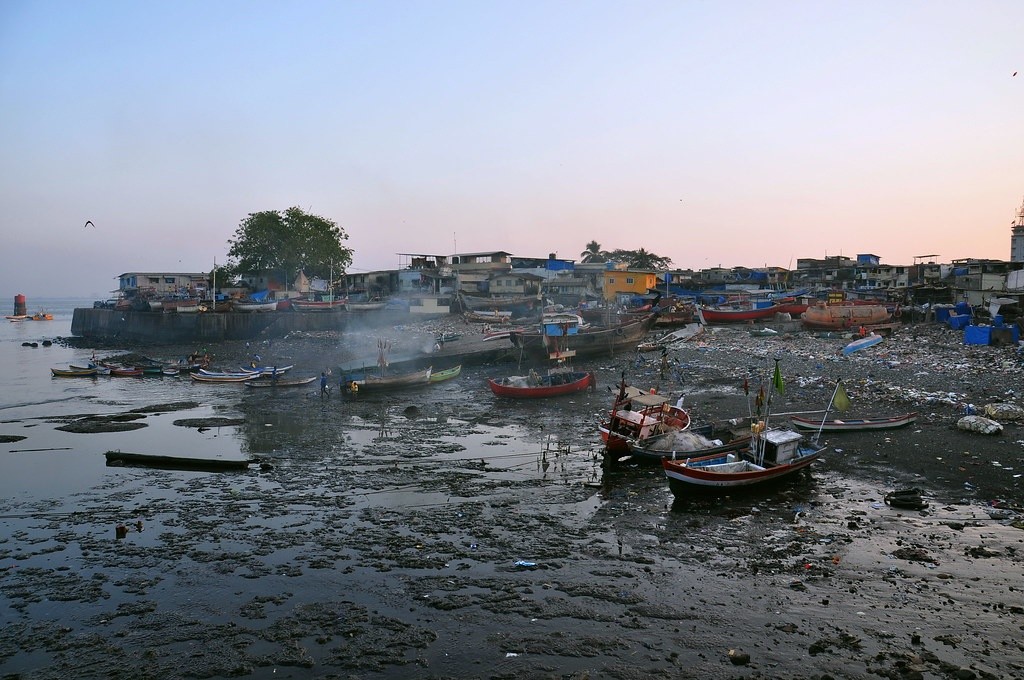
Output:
[743,378,765,416]
[831,384,851,413]
[542,333,551,348]
[536,283,543,301]
[773,366,784,395]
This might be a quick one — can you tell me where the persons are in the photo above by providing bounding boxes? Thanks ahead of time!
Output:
[254,354,261,363]
[869,328,875,337]
[441,334,445,346]
[858,325,867,338]
[272,365,279,385]
[320,372,329,399]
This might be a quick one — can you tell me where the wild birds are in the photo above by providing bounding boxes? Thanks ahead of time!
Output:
[84,221,95,227]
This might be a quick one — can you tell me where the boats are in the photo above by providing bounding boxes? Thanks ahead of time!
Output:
[749,328,783,336]
[94,257,395,313]
[789,410,918,435]
[50,357,295,383]
[843,334,884,358]
[343,363,462,391]
[623,412,771,469]
[598,373,691,457]
[435,335,462,343]
[32,308,54,321]
[660,424,829,500]
[482,329,527,342]
[508,305,661,365]
[488,366,597,398]
[243,376,318,387]
[468,283,894,364]
